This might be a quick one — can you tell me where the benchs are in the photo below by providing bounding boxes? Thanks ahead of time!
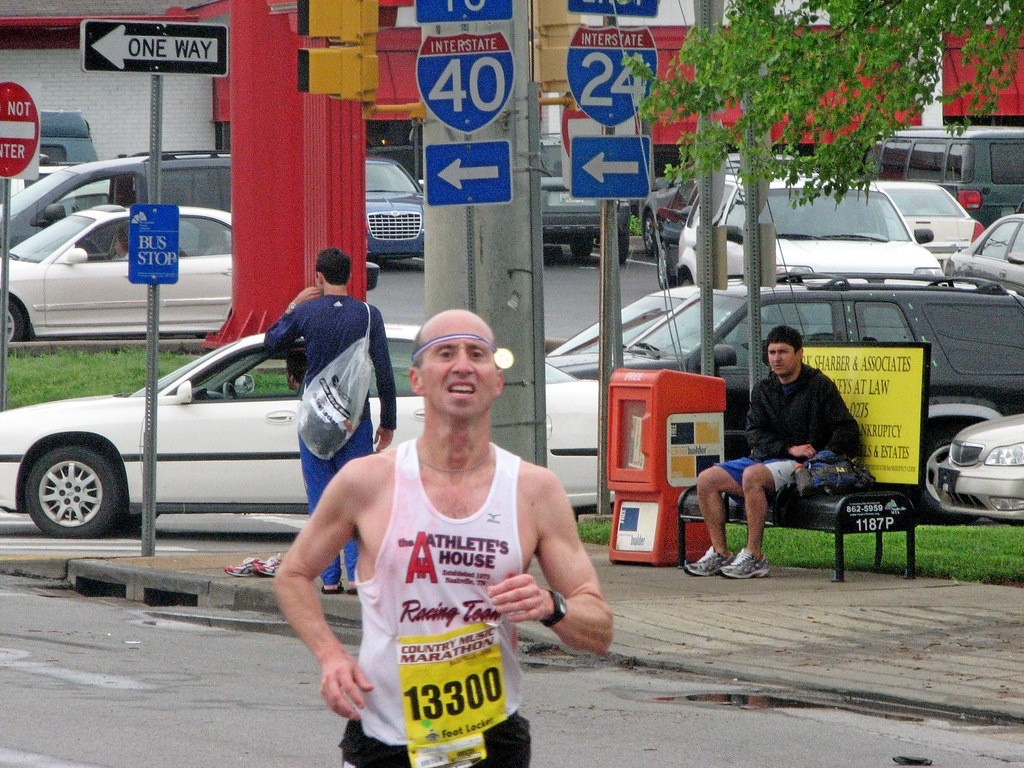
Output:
[676,483,918,582]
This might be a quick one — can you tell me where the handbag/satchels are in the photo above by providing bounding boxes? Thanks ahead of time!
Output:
[295,338,371,460]
[787,451,875,497]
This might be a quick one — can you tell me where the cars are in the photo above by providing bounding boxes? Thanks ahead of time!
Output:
[9,166,110,216]
[0,203,378,341]
[364,156,425,265]
[934,412,1024,526]
[641,153,795,290]
[869,180,985,267]
[945,214,1023,300]
[675,173,942,273]
[547,280,1016,357]
[1,323,618,539]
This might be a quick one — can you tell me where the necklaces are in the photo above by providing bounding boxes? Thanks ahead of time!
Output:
[419,443,492,474]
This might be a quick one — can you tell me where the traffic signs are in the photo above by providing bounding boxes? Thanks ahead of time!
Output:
[81,19,228,76]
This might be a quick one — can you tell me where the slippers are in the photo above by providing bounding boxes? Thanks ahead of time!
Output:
[321,580,344,593]
[347,589,358,594]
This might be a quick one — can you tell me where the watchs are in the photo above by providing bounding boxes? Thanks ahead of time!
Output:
[539,589,567,627]
[288,302,297,310]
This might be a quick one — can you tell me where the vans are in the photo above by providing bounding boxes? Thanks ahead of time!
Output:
[857,125,1024,229]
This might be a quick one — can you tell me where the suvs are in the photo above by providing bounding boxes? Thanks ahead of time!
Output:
[1,149,232,256]
[541,272,1024,525]
[539,133,631,263]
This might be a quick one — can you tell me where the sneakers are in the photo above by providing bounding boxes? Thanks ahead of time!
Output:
[253,553,283,576]
[719,548,770,578]
[683,546,735,576]
[224,557,266,576]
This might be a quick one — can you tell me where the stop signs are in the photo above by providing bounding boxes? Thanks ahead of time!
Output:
[0,75,41,181]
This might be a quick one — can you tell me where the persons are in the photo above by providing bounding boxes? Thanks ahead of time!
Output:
[109,227,130,261]
[684,325,861,578]
[266,249,395,595]
[273,310,614,768]
